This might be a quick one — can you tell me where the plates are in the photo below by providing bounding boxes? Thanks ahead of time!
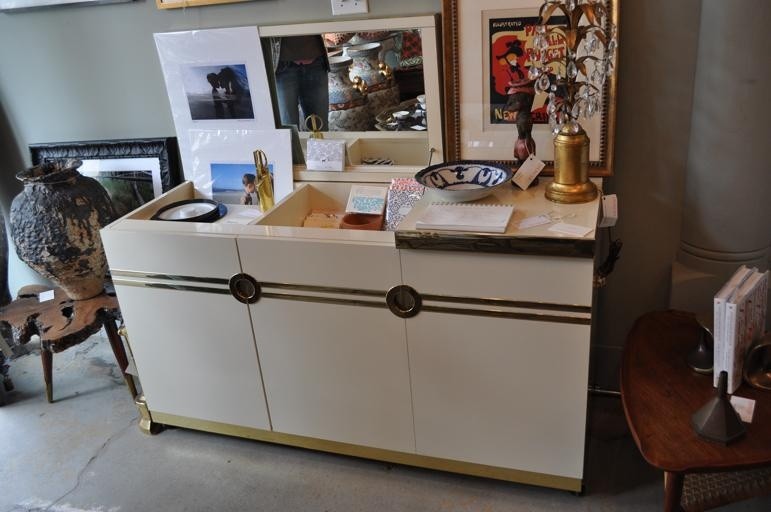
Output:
[414,159,514,203]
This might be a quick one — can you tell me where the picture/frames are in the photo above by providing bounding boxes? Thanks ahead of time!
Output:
[27,135,182,277]
[439,0,619,178]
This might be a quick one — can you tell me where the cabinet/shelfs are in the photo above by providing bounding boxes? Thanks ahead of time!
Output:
[99,176,603,494]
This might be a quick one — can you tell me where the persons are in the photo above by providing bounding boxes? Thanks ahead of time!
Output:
[216,67,252,118]
[266,34,332,131]
[208,74,234,119]
[238,172,257,205]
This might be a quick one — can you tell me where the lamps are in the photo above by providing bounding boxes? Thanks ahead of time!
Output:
[527,0,619,205]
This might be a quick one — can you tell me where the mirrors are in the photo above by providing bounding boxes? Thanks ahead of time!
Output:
[259,13,445,173]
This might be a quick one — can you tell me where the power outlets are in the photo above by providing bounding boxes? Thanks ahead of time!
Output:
[331,0,368,16]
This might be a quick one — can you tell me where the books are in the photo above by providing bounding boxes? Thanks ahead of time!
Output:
[709,260,771,394]
[415,201,518,234]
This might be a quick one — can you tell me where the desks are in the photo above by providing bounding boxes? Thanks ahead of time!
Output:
[319,31,399,57]
[0,284,141,404]
[618,308,771,512]
[374,98,426,133]
